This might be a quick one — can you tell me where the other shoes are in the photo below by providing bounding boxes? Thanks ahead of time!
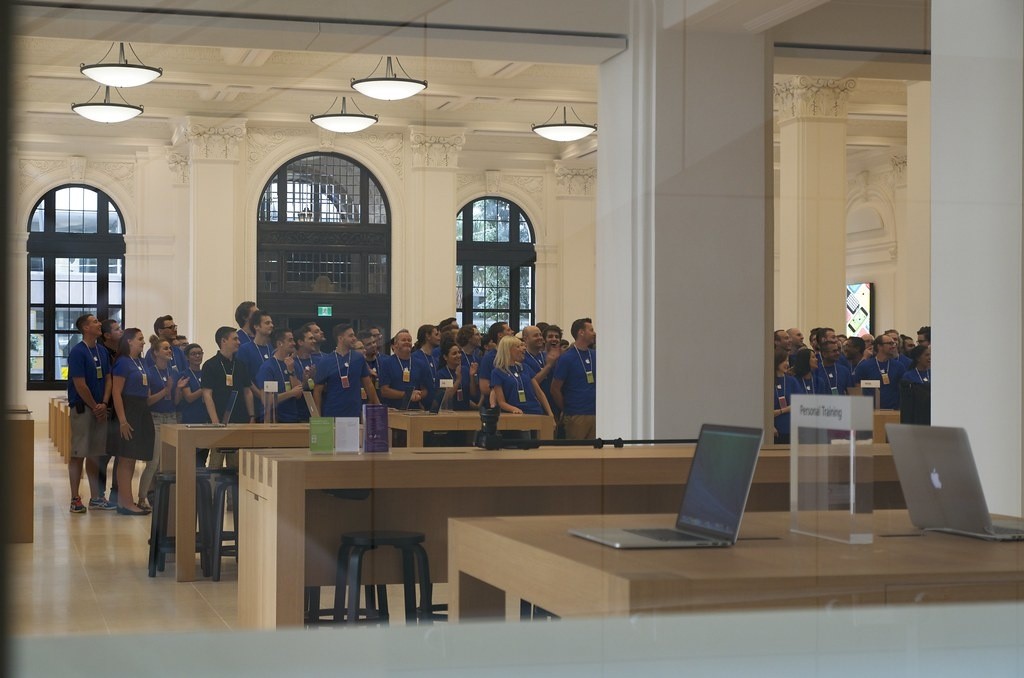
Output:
[109,493,117,506]
[148,492,154,506]
[122,507,151,515]
[136,503,150,510]
[117,503,123,512]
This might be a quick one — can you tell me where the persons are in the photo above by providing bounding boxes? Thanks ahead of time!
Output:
[68,314,116,513]
[101,301,931,514]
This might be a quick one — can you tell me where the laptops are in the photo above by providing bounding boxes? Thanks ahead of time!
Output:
[569,423,765,548]
[186,391,238,427]
[404,387,447,416]
[395,386,415,412]
[884,423,1024,541]
[303,392,319,417]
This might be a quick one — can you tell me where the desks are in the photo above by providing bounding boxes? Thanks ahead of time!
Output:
[238,445,898,629]
[160,424,390,577]
[446,509,1024,627]
[387,411,554,448]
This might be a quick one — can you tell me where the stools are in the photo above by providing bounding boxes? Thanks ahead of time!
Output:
[333,531,433,626]
[147,467,238,582]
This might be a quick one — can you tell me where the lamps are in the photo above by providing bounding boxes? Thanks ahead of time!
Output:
[530,106,598,142]
[310,97,379,133]
[80,43,164,87]
[350,56,428,101]
[70,87,144,125]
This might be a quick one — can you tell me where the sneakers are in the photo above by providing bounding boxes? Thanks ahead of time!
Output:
[89,498,117,510]
[70,496,86,513]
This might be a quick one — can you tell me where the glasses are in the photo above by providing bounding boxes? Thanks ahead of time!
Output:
[916,340,927,345]
[827,349,840,353]
[165,325,178,329]
[372,334,383,340]
[883,342,897,347]
[188,350,204,356]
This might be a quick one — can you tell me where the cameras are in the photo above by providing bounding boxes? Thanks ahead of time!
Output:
[473,405,502,450]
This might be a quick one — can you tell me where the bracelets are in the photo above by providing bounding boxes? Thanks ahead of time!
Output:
[250,415,256,419]
[102,402,108,406]
[287,370,296,375]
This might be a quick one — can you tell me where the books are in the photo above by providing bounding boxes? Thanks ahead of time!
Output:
[308,415,335,455]
[363,404,389,452]
[336,417,359,452]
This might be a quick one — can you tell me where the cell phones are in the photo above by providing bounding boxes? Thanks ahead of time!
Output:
[847,284,868,333]
